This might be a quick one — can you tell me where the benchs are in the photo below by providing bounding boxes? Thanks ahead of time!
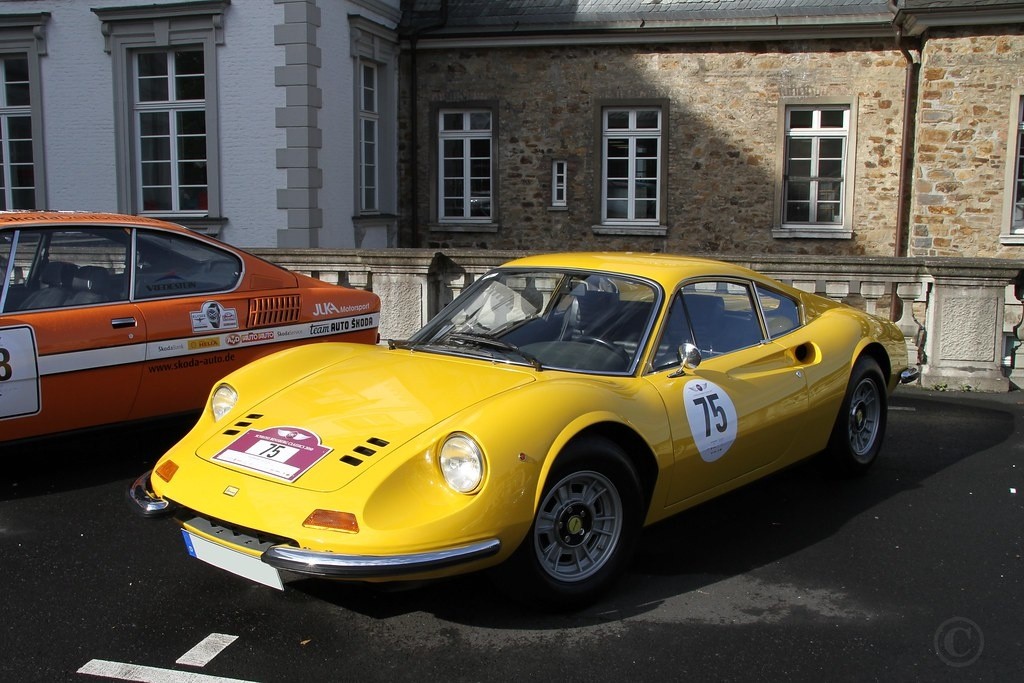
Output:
[621,300,794,353]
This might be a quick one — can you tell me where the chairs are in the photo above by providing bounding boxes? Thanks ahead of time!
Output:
[558,291,725,364]
[19,262,109,312]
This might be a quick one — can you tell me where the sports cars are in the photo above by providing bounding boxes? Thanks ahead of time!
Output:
[134,251,921,598]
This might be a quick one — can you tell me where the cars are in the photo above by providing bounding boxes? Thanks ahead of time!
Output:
[0,212,381,441]
